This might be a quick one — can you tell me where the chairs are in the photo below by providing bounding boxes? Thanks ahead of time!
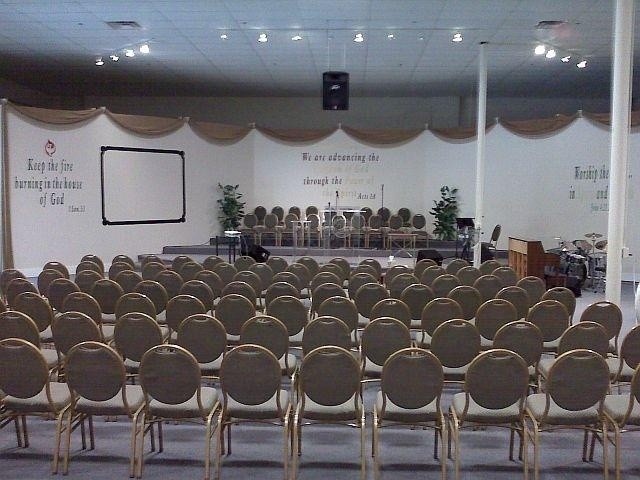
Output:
[113,312,172,424]
[432,274,461,297]
[0,311,65,420]
[479,321,541,430]
[329,257,353,280]
[495,285,531,321]
[537,321,611,432]
[215,343,292,480]
[43,262,70,283]
[390,273,419,299]
[473,274,504,301]
[284,263,311,288]
[203,256,224,270]
[387,233,419,257]
[590,364,640,480]
[273,272,309,294]
[537,287,576,326]
[318,296,360,350]
[112,255,135,269]
[242,202,429,252]
[319,265,345,281]
[266,281,300,308]
[116,293,157,327]
[113,270,142,292]
[266,256,288,274]
[142,262,167,280]
[358,316,420,429]
[6,278,39,306]
[165,294,211,385]
[63,292,115,345]
[414,319,481,431]
[448,349,529,480]
[38,269,68,298]
[220,280,256,309]
[180,262,205,283]
[480,260,502,275]
[76,261,104,279]
[312,272,350,297]
[132,280,173,353]
[261,296,312,346]
[516,276,547,308]
[520,349,610,480]
[386,265,412,289]
[233,271,265,315]
[400,284,435,328]
[52,311,113,422]
[312,283,346,320]
[75,270,103,292]
[0,297,10,313]
[421,265,447,284]
[216,294,263,341]
[349,273,378,301]
[491,267,516,291]
[0,338,86,474]
[445,258,468,275]
[297,257,320,281]
[0,389,22,447]
[177,314,240,425]
[87,279,125,314]
[179,280,213,314]
[414,259,438,282]
[193,270,224,306]
[475,299,517,350]
[359,258,382,280]
[212,263,236,282]
[137,344,221,480]
[605,324,640,394]
[235,257,256,274]
[373,347,446,480]
[172,256,193,271]
[415,297,464,348]
[290,346,365,480]
[12,292,52,343]
[455,266,481,286]
[248,263,273,290]
[109,262,134,282]
[141,255,164,270]
[155,270,184,300]
[584,301,623,357]
[481,224,502,263]
[526,300,570,358]
[239,315,299,425]
[0,269,29,302]
[354,283,391,327]
[356,265,381,283]
[446,285,482,326]
[298,316,362,423]
[80,255,105,274]
[63,341,155,478]
[371,299,411,333]
[46,278,81,311]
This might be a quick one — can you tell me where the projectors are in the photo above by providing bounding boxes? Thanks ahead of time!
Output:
[224,230,241,237]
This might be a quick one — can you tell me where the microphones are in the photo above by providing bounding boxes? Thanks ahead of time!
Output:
[336,190,339,198]
[328,202,330,207]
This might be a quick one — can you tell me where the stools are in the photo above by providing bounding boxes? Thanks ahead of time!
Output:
[594,266,605,293]
[568,275,582,298]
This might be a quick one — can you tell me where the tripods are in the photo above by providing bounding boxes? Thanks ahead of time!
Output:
[460,233,473,263]
[582,253,607,294]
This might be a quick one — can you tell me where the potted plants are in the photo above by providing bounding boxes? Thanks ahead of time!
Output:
[216,183,247,243]
[428,184,460,241]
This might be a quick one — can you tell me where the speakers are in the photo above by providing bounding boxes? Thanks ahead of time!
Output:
[323,73,350,110]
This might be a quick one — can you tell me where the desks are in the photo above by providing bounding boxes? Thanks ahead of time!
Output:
[507,236,560,286]
